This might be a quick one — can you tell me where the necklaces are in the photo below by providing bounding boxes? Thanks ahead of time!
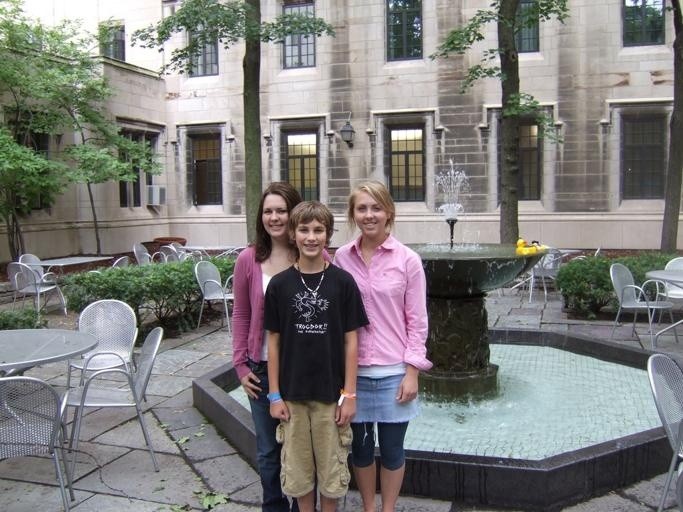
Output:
[297,259,326,298]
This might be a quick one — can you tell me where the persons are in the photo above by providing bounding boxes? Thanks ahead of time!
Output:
[261,200,369,511]
[231,182,331,511]
[331,180,433,512]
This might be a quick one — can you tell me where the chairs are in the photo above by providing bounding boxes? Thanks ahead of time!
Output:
[18,253,62,309]
[60,326,163,482]
[194,261,234,337]
[68,299,147,404]
[7,262,68,322]
[646,354,682,512]
[0,375,76,512]
[527,249,570,303]
[572,246,601,262]
[658,256,683,326]
[609,262,678,352]
[102,229,248,274]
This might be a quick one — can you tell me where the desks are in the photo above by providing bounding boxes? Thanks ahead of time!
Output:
[0,330,99,446]
[644,268,683,333]
[26,256,114,312]
[509,250,582,294]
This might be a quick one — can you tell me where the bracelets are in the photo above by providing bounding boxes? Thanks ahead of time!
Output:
[267,393,283,403]
[340,389,357,400]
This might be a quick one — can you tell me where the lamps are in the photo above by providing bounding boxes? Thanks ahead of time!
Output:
[339,112,355,147]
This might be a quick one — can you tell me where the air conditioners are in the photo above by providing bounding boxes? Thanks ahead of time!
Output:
[145,183,167,205]
